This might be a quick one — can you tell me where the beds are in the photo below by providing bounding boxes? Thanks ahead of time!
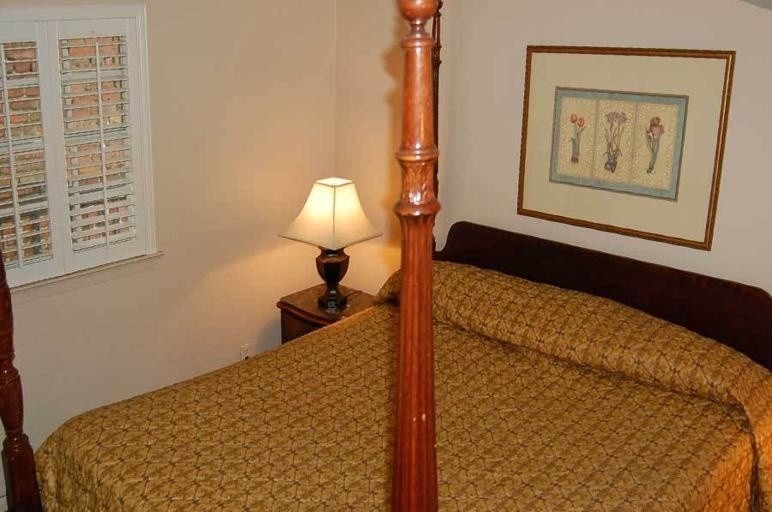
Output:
[0,0,772,512]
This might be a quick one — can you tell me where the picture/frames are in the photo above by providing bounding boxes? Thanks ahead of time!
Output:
[517,46,737,251]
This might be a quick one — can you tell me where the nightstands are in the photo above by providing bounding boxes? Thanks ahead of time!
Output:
[277,283,377,345]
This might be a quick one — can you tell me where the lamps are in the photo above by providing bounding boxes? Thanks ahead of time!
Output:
[278,176,382,308]
